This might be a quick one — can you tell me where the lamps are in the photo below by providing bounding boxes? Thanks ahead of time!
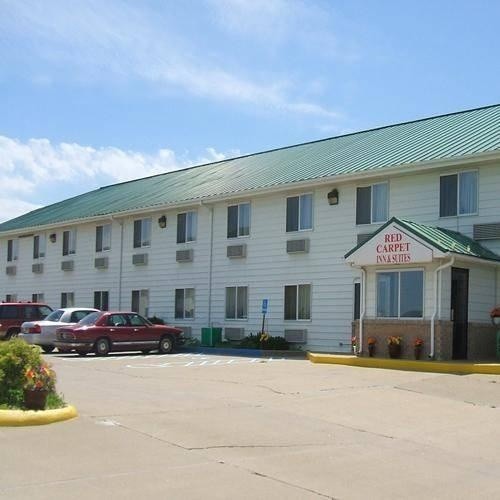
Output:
[49,233,56,243]
[327,187,339,205]
[158,215,166,228]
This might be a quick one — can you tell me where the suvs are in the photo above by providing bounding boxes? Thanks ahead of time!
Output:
[1,301,54,343]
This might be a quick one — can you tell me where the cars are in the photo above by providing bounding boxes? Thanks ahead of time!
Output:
[18,308,102,354]
[52,310,183,357]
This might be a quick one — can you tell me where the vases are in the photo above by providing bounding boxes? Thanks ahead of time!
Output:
[388,345,400,359]
[23,390,48,410]
[353,346,358,355]
[368,343,374,357]
[414,347,421,360]
[492,317,500,325]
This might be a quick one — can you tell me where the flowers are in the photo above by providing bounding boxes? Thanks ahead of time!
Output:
[414,338,424,346]
[367,336,377,344]
[352,336,358,345]
[491,308,500,317]
[25,365,52,390]
[384,335,404,344]
